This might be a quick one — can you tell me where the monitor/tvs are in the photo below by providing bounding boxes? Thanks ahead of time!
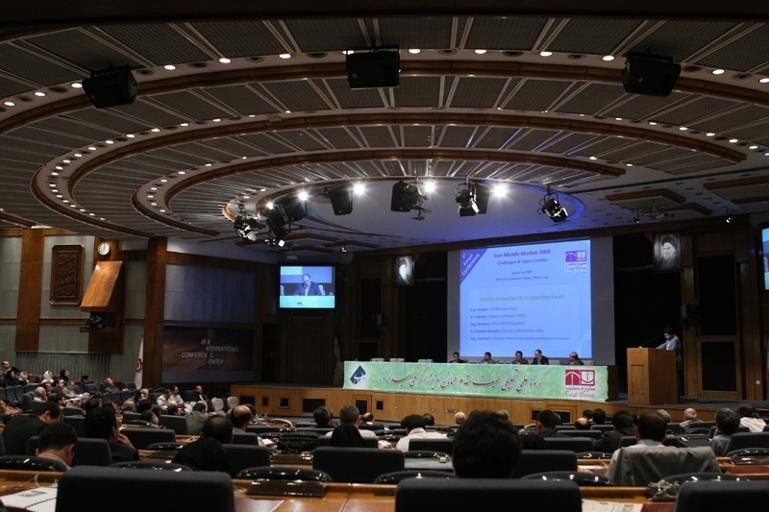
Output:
[277,262,337,312]
[759,223,769,295]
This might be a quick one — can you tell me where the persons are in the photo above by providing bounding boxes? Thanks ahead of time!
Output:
[295,273,323,295]
[660,240,678,267]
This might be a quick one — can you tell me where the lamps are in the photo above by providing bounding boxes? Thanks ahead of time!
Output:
[234,178,366,246]
[78,66,142,111]
[390,180,436,221]
[345,45,403,90]
[540,186,568,223]
[622,53,682,98]
[456,180,508,216]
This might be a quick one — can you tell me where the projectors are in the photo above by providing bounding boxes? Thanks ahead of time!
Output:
[246,229,276,242]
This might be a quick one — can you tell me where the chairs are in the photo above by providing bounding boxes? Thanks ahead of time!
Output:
[1,357,768,512]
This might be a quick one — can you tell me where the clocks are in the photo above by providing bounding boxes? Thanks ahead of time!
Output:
[98,241,110,256]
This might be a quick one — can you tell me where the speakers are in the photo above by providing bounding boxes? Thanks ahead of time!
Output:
[623,57,680,96]
[391,183,411,212]
[282,197,304,222]
[346,51,400,88]
[82,71,138,108]
[266,206,289,232]
[329,189,352,215]
[475,184,488,213]
